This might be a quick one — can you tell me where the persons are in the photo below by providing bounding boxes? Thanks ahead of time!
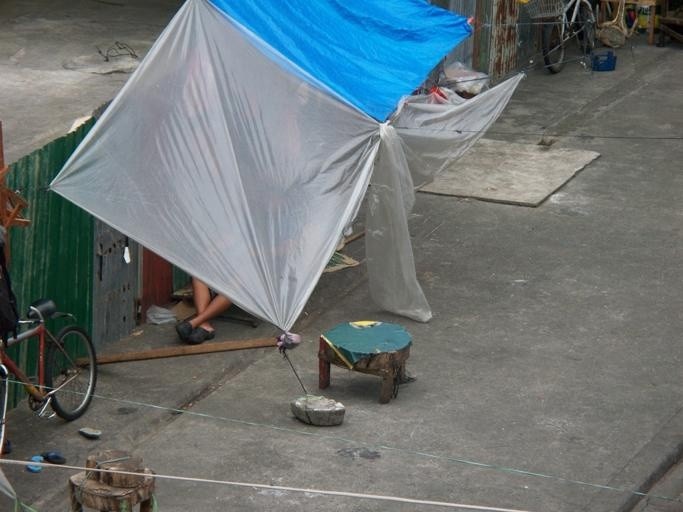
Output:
[176,275,231,346]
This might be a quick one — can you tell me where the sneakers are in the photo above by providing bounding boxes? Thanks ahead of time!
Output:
[175,314,215,344]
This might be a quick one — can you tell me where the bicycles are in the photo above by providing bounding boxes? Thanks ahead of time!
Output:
[527,0,596,74]
[1,299,98,451]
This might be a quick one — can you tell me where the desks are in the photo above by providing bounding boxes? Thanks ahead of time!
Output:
[318,319,413,403]
